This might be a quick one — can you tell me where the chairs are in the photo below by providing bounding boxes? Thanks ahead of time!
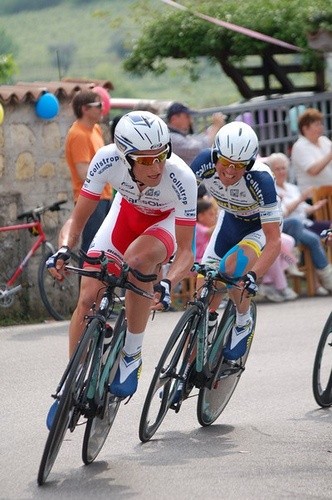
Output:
[170,185,332,306]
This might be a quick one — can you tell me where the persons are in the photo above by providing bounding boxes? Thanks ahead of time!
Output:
[66,89,115,288]
[189,121,281,361]
[292,108,332,220]
[48,117,197,431]
[110,116,331,303]
[165,104,225,168]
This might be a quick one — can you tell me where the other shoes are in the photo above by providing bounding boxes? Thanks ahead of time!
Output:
[316,264,332,296]
[286,265,303,277]
[280,288,297,299]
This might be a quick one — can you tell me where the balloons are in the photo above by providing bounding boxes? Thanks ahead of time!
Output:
[36,94,59,119]
[93,86,111,116]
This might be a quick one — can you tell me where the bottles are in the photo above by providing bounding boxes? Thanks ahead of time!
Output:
[86,324,114,360]
[207,309,219,349]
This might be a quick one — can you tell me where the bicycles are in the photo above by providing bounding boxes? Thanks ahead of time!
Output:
[139,254,258,444]
[36,248,166,487]
[312,310,332,408]
[0,199,83,322]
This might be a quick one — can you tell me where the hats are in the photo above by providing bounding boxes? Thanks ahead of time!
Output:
[167,103,197,117]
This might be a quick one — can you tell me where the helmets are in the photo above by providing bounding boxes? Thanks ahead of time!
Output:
[210,120,258,171]
[114,110,172,171]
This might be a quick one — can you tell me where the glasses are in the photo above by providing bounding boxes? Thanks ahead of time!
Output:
[216,152,251,169]
[89,102,102,109]
[127,144,170,166]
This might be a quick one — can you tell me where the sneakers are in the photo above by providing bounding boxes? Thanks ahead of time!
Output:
[223,318,252,361]
[47,399,68,429]
[159,388,182,402]
[109,348,143,396]
